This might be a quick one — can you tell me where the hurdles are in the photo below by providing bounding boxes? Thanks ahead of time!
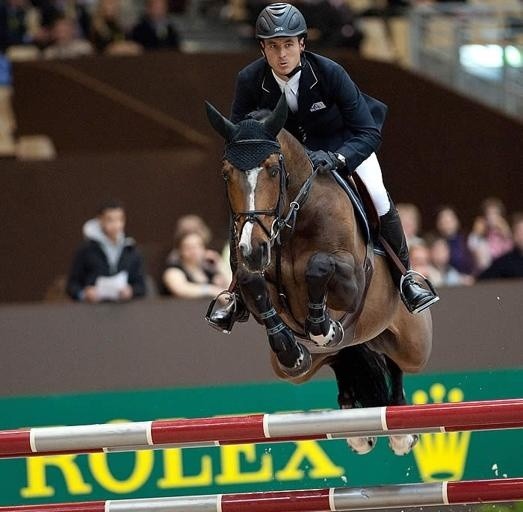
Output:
[0,399,523,512]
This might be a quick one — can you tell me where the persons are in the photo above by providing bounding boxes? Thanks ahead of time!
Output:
[421,205,477,276]
[205,0,440,336]
[64,196,146,306]
[466,195,513,269]
[163,213,212,268]
[410,239,477,287]
[0,51,58,162]
[392,202,428,252]
[156,232,233,300]
[474,208,523,284]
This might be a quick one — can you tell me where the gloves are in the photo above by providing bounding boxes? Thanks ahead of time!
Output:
[308,151,343,174]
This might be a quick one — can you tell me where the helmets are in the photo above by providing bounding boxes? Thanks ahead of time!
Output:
[255,3,308,40]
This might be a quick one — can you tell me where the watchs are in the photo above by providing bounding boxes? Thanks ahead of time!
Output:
[334,152,347,167]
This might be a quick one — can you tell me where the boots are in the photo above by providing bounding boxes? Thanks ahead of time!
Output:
[209,218,249,329]
[377,192,434,310]
